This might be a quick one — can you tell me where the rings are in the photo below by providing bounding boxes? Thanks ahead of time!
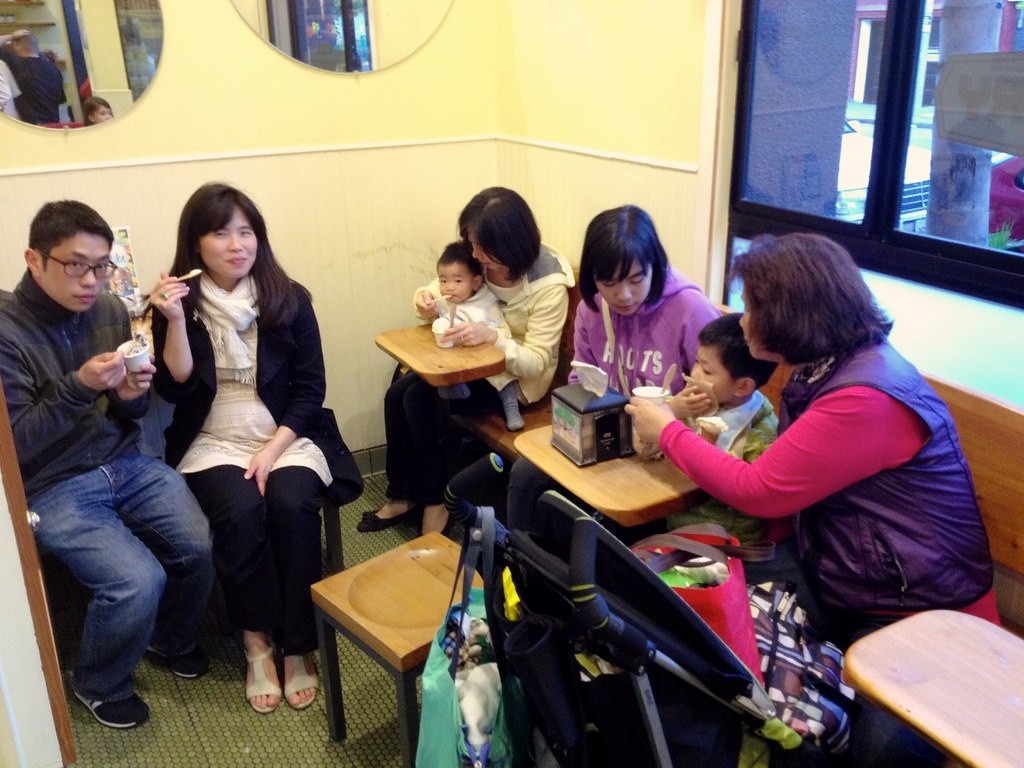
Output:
[161,293,168,299]
[461,338,465,343]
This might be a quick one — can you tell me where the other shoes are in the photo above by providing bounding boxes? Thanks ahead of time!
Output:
[357,507,420,532]
[421,511,453,537]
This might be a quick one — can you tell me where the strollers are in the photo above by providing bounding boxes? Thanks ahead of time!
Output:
[443,453,821,768]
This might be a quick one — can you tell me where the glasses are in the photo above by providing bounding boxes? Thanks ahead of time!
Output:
[33,248,118,278]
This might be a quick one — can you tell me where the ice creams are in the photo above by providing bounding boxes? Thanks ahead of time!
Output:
[432,317,450,333]
[127,331,148,356]
[696,417,726,445]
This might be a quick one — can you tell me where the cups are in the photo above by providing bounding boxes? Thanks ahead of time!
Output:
[632,386,670,405]
[431,327,459,348]
[117,340,152,373]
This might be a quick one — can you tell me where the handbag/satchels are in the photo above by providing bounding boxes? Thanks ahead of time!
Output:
[414,506,511,768]
[629,522,855,754]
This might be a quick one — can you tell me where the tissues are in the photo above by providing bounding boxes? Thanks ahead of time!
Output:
[549,361,638,469]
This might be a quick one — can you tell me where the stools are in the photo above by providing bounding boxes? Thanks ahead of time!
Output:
[311,532,511,768]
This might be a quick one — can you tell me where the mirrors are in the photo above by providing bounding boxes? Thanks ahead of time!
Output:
[228,0,455,72]
[0,0,164,130]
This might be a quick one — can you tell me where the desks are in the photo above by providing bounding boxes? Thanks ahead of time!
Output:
[375,324,506,388]
[842,609,1024,768]
[511,403,733,526]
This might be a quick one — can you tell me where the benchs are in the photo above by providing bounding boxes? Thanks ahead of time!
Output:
[706,300,1024,638]
[399,273,1024,645]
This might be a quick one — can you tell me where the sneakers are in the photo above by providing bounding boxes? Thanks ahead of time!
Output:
[69,671,150,728]
[147,640,213,678]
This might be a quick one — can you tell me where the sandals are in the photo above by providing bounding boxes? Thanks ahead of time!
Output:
[243,635,320,713]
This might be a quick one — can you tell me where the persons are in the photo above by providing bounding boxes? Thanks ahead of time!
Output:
[0,201,215,728]
[624,233,1003,768]
[506,205,722,535]
[658,312,782,553]
[1,30,113,130]
[354,187,576,537]
[436,240,526,431]
[150,182,365,715]
